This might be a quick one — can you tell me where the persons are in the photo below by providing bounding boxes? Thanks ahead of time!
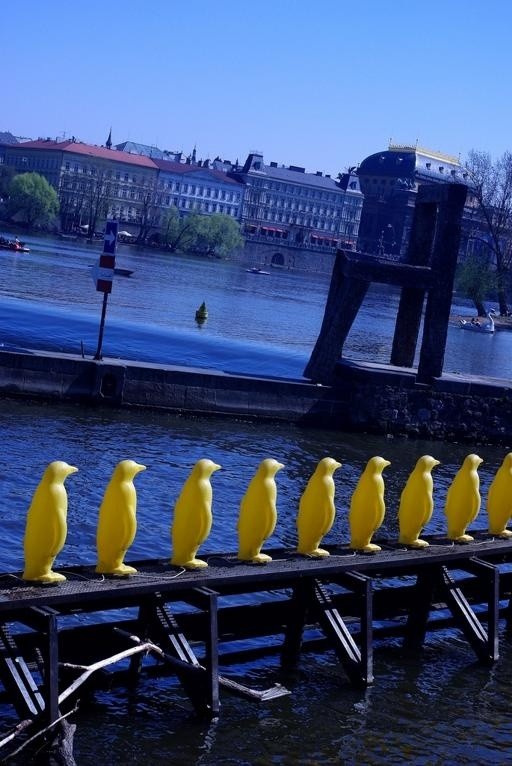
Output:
[475,319,480,325]
[470,318,475,325]
[15,236,20,242]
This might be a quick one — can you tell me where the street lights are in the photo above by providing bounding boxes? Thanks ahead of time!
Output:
[343,201,363,250]
[246,182,268,234]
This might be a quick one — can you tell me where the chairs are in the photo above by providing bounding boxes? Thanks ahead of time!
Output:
[298,176,472,391]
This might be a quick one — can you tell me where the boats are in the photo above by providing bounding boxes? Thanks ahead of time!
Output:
[246,267,270,275]
[0,241,30,251]
[459,307,495,334]
[88,264,134,276]
